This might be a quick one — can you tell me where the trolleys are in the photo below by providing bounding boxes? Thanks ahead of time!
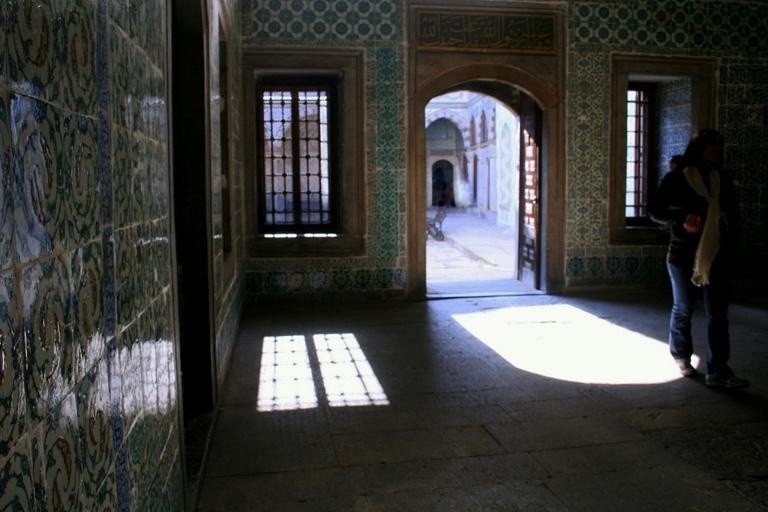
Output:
[426,206,447,241]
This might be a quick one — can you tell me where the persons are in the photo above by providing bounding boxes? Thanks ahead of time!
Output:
[641,128,751,390]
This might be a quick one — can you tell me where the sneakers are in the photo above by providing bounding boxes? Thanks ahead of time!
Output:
[677,362,696,377]
[705,367,750,388]
